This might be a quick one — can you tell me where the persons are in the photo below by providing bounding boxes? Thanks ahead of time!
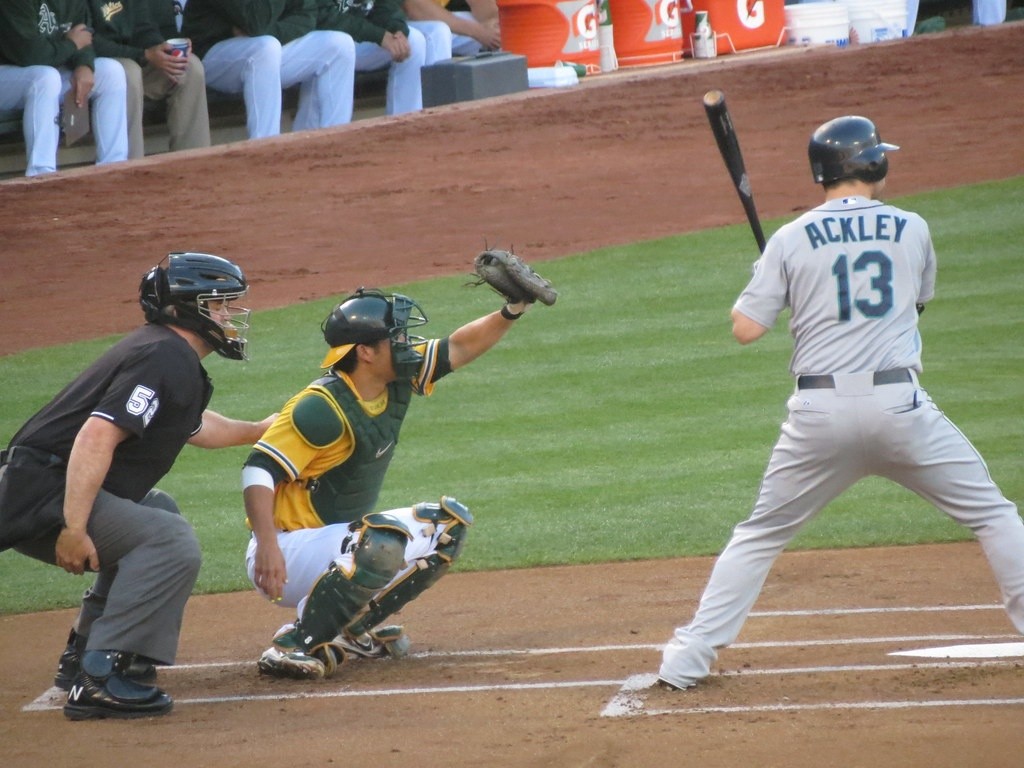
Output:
[657,115,1024,693]
[241,250,558,680]
[0,252,282,721]
[0,0,501,179]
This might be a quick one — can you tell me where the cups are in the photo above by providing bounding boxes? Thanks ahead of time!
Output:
[554,60,586,76]
[694,10,715,59]
[166,39,188,70]
[596,0,615,73]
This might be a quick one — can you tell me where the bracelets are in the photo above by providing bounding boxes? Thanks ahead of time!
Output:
[501,306,523,320]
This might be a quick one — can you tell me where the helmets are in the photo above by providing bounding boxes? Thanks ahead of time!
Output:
[138,252,250,363]
[321,294,400,368]
[807,116,900,182]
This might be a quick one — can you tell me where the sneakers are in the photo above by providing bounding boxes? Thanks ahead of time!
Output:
[257,646,325,679]
[54,628,156,689]
[63,649,174,718]
[332,634,389,659]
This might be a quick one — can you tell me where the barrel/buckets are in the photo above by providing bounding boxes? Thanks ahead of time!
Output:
[680,0,787,54]
[608,0,685,68]
[495,0,600,76]
[842,0,909,47]
[784,2,849,51]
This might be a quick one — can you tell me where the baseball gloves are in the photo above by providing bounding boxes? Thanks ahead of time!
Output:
[474,248,556,309]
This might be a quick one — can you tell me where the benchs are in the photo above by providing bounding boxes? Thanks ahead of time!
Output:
[1,67,391,144]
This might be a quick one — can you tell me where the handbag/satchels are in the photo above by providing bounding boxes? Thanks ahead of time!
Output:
[0,447,67,552]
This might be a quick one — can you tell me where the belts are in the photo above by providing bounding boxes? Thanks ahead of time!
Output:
[798,368,913,389]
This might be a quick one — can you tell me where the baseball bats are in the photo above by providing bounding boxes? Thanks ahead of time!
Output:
[702,88,767,255]
